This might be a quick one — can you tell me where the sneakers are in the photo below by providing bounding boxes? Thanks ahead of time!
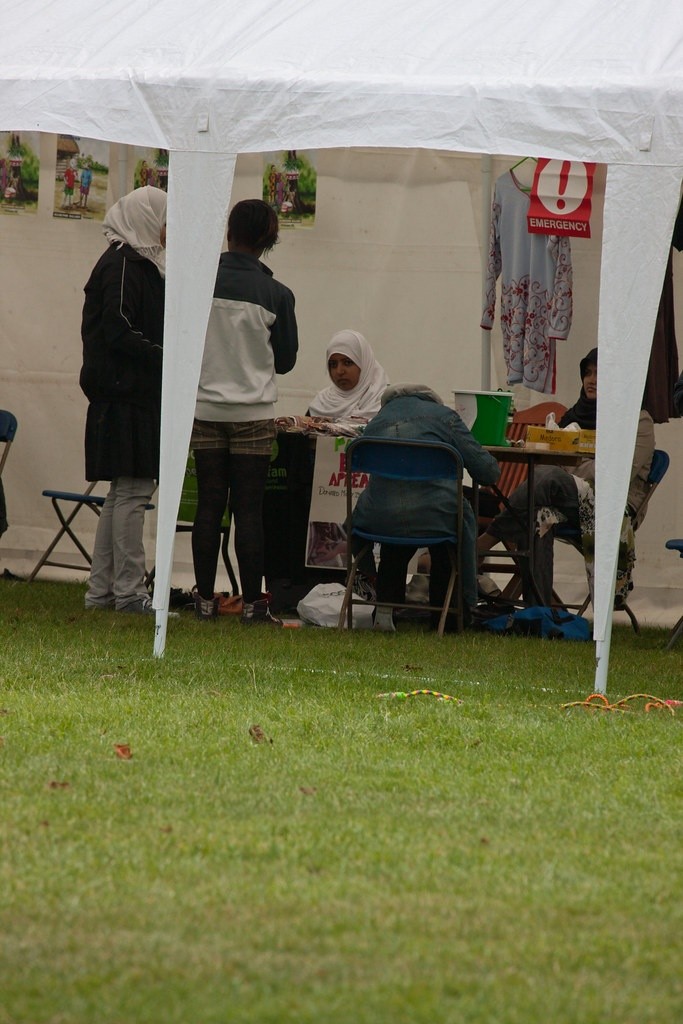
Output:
[241,599,280,626]
[194,591,223,621]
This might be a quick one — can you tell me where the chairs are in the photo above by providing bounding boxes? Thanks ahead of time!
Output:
[25,480,160,590]
[335,435,465,640]
[0,408,18,477]
[144,449,240,598]
[496,449,670,634]
[477,401,568,605]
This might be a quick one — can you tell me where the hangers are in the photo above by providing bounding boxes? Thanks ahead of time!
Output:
[509,156,539,192]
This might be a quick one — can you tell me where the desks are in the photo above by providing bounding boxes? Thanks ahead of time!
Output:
[276,426,595,626]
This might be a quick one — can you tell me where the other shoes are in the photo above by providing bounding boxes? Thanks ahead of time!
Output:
[417,547,484,574]
[120,597,180,620]
[372,584,396,632]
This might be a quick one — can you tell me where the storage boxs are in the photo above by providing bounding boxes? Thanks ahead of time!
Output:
[526,426,595,453]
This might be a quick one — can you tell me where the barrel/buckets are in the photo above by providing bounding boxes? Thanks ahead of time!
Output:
[450,389,515,446]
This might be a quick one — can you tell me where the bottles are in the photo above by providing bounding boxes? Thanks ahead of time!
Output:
[279,619,306,628]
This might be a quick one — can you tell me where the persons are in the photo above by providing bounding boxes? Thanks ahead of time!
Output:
[78,185,168,614]
[306,330,389,417]
[478,348,657,609]
[343,382,500,633]
[192,199,299,627]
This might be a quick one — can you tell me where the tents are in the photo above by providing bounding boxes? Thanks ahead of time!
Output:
[0,0,683,694]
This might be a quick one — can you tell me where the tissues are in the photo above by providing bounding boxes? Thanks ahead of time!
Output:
[525,412,596,453]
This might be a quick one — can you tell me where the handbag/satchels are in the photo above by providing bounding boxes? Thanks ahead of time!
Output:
[479,603,591,641]
[297,580,376,631]
